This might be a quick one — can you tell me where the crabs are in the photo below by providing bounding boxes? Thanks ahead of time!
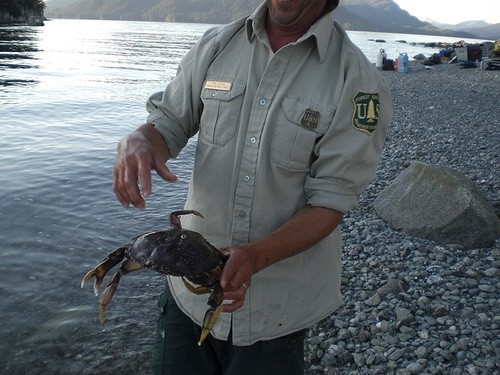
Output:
[80,209,228,346]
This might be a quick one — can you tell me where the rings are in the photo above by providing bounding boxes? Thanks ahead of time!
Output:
[242,283,249,291]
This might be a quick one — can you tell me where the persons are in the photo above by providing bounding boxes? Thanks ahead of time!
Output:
[112,0,391,374]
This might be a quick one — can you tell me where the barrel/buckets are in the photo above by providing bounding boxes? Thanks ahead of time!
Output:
[398,53,408,72]
[394,56,400,71]
[376,49,386,67]
[382,59,393,70]
